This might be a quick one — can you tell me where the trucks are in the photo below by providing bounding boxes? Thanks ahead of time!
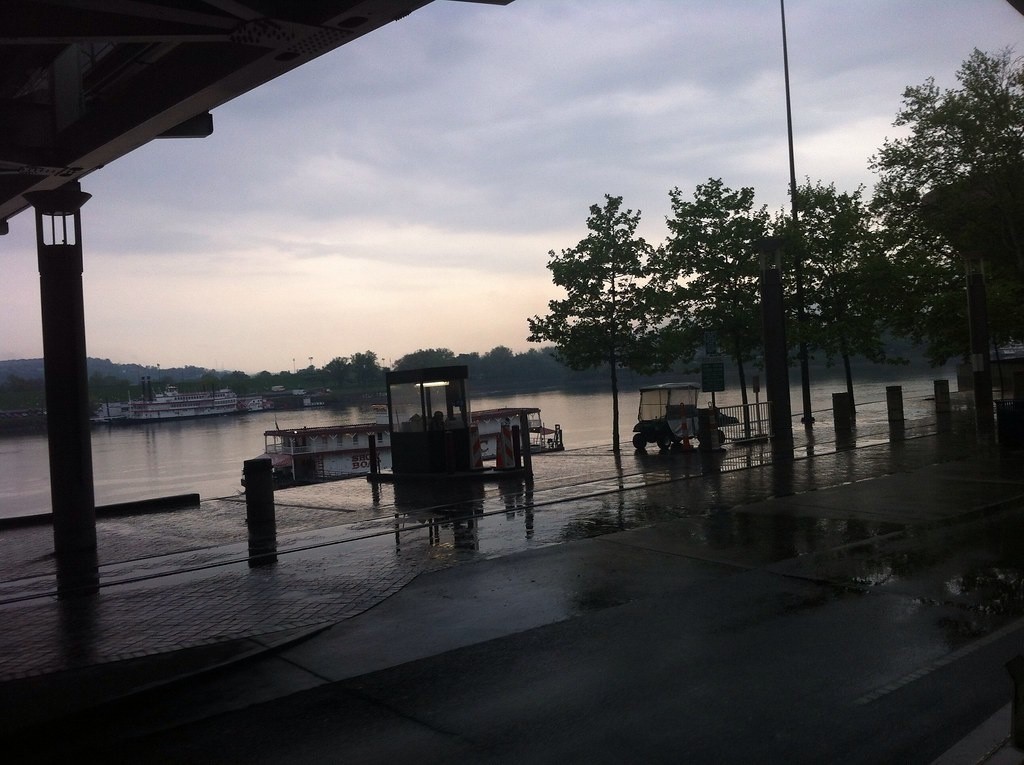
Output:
[293,390,306,396]
[272,386,285,392]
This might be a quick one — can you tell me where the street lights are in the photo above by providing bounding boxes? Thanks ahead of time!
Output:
[23,190,101,593]
[962,248,998,447]
[755,236,794,461]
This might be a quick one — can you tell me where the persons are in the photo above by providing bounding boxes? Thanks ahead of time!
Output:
[410,411,446,432]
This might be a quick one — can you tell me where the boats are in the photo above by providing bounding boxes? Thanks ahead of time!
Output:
[240,407,566,494]
[247,398,274,412]
[90,375,253,424]
